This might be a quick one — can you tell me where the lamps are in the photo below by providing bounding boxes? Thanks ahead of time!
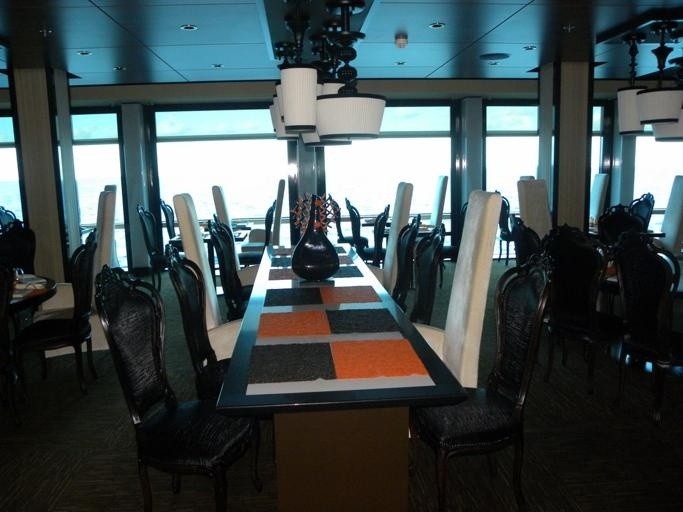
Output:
[604,11,683,142]
[267,0,388,148]
